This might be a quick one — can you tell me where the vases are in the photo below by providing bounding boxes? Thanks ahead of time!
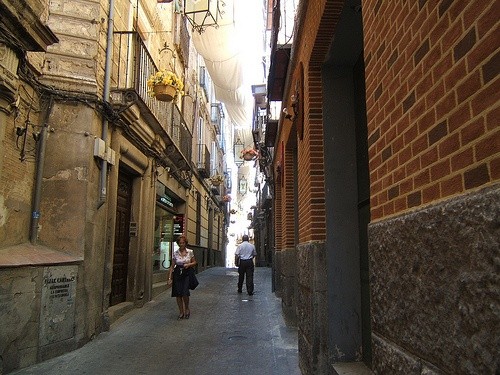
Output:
[152,83,176,101]
[244,153,255,160]
[213,182,220,187]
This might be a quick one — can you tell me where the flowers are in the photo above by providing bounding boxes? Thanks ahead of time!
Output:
[240,149,258,159]
[147,70,184,102]
[210,176,223,182]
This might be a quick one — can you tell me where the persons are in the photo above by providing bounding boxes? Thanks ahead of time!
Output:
[167,236,197,320]
[235,235,257,296]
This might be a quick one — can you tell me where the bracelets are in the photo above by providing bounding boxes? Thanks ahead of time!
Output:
[169,278,171,280]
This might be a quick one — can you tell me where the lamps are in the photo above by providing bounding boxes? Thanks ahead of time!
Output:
[276,161,281,174]
[283,108,293,122]
[290,95,299,117]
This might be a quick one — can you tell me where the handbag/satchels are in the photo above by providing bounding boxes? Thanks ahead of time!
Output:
[189,268,199,290]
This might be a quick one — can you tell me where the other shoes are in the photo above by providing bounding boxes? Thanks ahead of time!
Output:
[185,310,190,319]
[177,314,184,320]
